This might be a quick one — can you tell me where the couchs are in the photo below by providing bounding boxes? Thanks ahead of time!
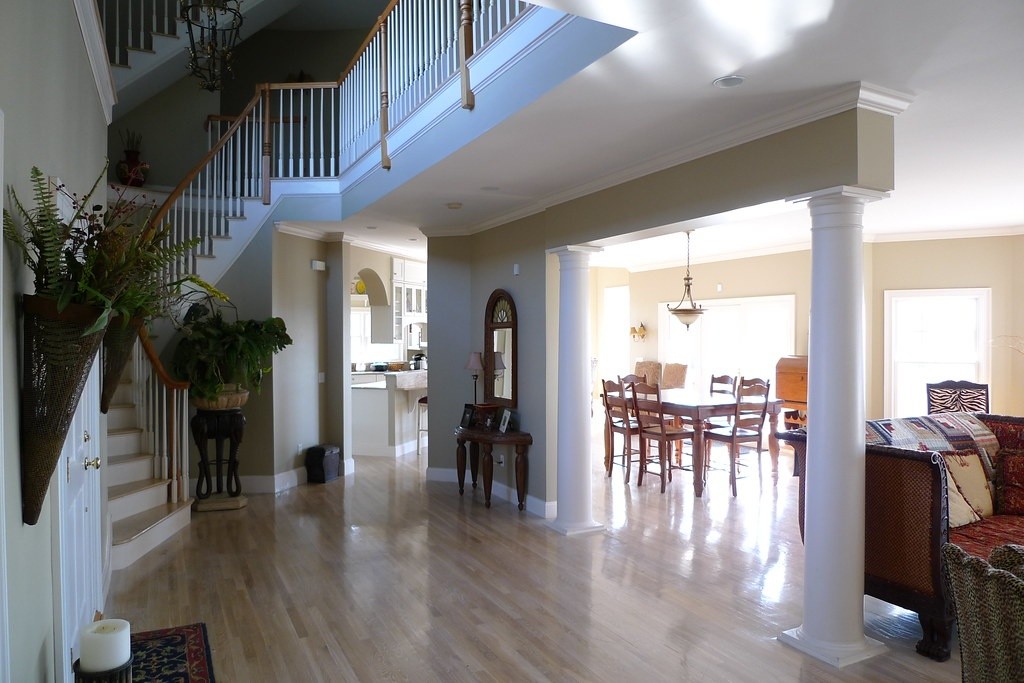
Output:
[942,542,1024,683]
[774,411,1024,664]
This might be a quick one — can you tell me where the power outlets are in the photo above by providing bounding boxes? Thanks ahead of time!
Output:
[500,455,504,467]
[298,443,303,456]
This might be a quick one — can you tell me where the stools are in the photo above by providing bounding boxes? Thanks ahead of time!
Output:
[417,396,427,455]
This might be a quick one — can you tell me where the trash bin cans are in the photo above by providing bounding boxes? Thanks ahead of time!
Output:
[305,444,340,483]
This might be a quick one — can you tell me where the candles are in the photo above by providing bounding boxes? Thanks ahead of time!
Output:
[79,619,131,672]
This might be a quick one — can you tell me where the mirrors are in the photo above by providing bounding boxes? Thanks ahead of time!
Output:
[484,288,517,408]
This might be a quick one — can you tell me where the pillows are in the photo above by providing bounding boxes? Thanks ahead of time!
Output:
[994,447,1024,517]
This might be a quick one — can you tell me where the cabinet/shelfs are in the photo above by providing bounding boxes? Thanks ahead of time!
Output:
[351,374,387,385]
[371,257,428,344]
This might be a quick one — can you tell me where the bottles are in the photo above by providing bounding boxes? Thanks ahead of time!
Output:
[350,280,366,295]
[414,357,428,370]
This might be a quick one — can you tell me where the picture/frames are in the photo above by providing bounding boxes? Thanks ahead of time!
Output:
[499,409,514,433]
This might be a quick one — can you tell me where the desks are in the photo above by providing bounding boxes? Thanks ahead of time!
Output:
[190,409,248,513]
[600,387,785,498]
[453,424,532,513]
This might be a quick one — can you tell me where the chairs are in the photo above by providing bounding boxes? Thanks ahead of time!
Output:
[703,376,770,496]
[635,361,662,387]
[618,374,673,465]
[927,379,989,415]
[601,378,664,485]
[705,376,769,476]
[677,373,737,467]
[661,363,688,390]
[629,381,695,494]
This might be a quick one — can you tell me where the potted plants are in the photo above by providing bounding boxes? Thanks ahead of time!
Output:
[169,295,294,410]
[3,156,241,527]
[116,128,148,188]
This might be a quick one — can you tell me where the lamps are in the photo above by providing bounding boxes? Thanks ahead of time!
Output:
[514,264,520,280]
[667,229,708,331]
[173,0,246,93]
[494,352,506,382]
[465,352,484,404]
[629,322,648,343]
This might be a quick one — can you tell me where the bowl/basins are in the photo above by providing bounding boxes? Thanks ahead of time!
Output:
[386,363,404,370]
[370,364,388,372]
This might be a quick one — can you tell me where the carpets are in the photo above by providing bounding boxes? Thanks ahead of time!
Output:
[130,622,216,683]
